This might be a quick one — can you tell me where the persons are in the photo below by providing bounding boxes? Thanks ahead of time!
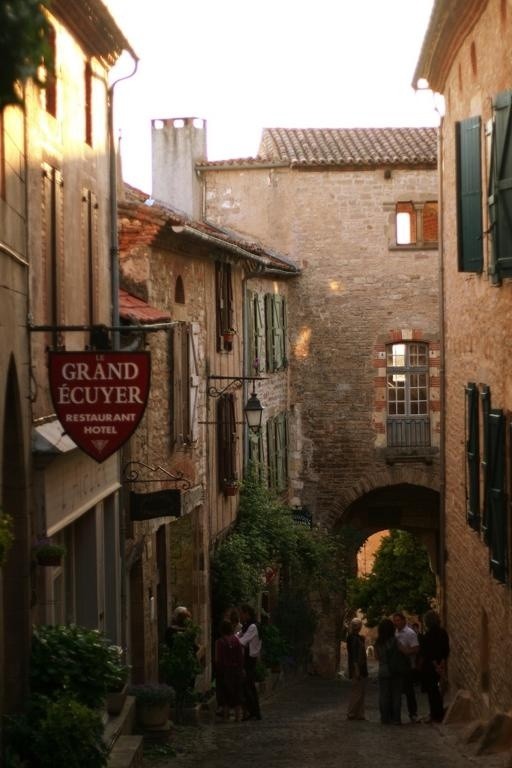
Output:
[375,612,449,725]
[165,604,262,721]
[347,618,368,720]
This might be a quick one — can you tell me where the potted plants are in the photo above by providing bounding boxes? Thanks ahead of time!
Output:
[129,681,178,733]
[219,474,241,497]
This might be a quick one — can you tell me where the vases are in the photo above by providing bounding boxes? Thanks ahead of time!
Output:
[177,702,201,726]
[104,683,129,714]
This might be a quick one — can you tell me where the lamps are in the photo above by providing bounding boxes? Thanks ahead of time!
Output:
[208,373,271,435]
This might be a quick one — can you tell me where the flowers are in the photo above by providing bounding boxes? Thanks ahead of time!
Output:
[101,645,129,692]
[158,605,206,706]
